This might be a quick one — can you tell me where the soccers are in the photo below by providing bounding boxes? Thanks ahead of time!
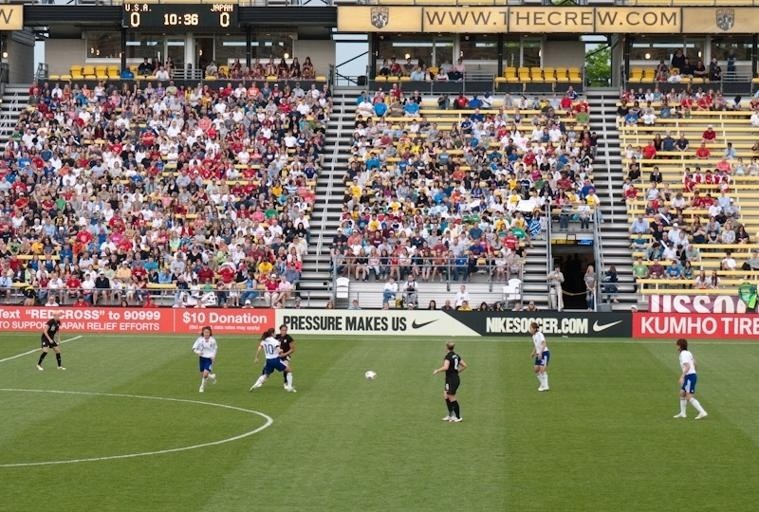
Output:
[365,370,377,379]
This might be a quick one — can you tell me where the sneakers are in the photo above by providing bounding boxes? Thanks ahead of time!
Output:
[673,410,688,418]
[538,386,550,392]
[440,414,463,422]
[199,384,207,393]
[56,366,66,371]
[695,409,709,420]
[211,373,217,384]
[249,382,262,391]
[37,364,44,371]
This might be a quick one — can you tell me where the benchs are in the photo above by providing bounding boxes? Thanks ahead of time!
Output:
[617,98,757,305]
[356,101,592,156]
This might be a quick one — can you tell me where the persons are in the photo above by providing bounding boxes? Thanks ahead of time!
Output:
[433,343,468,424]
[274,325,295,391]
[530,323,551,391]
[192,327,218,393]
[35,310,67,372]
[673,339,707,420]
[248,328,297,393]
[614,47,759,314]
[603,266,620,303]
